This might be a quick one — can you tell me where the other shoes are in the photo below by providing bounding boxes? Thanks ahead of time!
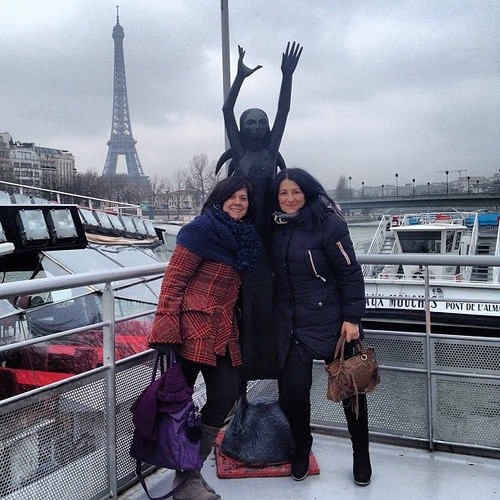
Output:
[291,421,313,481]
[352,435,372,485]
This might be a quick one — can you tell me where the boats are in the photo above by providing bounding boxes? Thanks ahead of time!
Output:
[0,180,172,498]
[361,212,500,316]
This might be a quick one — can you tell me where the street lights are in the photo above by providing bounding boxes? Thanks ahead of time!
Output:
[427,182,430,188]
[349,177,352,199]
[476,180,479,193]
[381,185,384,196]
[467,176,470,193]
[412,179,415,194]
[362,182,364,197]
[445,171,449,194]
[395,174,399,196]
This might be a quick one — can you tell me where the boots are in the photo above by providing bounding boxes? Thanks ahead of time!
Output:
[173,422,222,500]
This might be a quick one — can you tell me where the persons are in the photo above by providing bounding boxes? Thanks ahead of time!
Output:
[148,176,252,500]
[215,39,303,409]
[270,167,373,486]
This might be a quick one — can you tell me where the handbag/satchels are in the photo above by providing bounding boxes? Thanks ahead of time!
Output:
[325,331,380,418]
[130,343,202,500]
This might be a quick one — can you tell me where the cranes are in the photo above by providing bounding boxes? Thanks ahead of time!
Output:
[436,169,468,176]
